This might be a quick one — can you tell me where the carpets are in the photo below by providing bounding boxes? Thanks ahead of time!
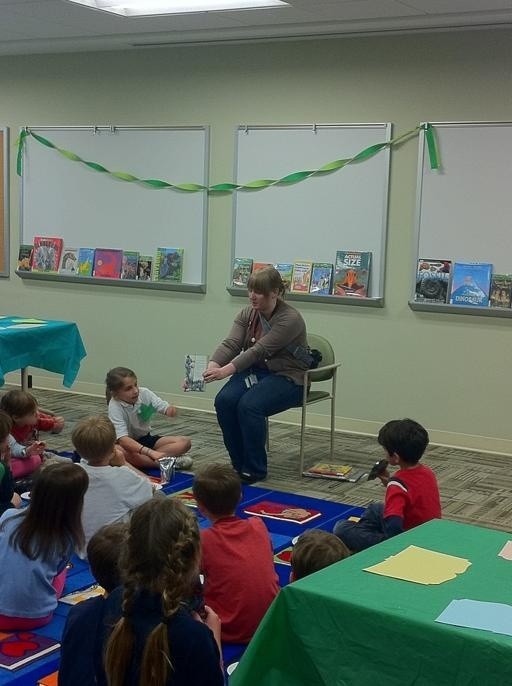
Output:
[0,449,368,686]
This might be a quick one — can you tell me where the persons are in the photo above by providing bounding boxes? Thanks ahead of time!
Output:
[289,528,351,583]
[183,266,312,486]
[333,417,441,552]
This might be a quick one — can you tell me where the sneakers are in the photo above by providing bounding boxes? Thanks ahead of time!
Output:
[239,471,262,486]
[173,455,192,469]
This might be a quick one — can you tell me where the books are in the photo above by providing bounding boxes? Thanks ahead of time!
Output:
[18,237,184,282]
[302,462,365,482]
[415,258,512,309]
[184,355,209,392]
[232,251,372,297]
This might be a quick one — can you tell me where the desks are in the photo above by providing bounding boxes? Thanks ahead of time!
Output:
[285,517,511,686]
[0,314,76,391]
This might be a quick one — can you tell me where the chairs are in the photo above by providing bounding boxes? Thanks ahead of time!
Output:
[266,334,342,480]
